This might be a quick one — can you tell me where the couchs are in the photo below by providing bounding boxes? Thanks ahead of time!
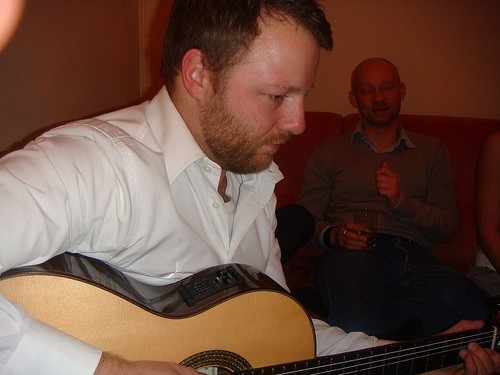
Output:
[274,112,500,278]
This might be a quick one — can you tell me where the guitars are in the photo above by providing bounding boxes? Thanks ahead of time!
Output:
[0,251,500,375]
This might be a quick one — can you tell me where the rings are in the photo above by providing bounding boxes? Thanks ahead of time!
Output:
[343,230,347,236]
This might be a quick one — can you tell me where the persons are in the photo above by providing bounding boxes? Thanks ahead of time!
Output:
[0,0,500,375]
[295,57,496,342]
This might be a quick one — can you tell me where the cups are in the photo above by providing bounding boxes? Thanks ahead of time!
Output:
[346,206,375,249]
